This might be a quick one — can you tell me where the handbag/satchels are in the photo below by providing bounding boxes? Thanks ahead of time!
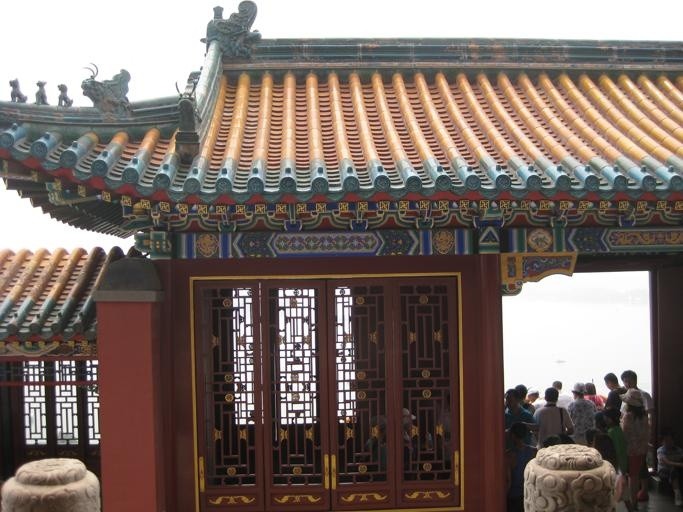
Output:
[560,434,574,443]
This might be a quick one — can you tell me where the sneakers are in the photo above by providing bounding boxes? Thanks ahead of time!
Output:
[637,490,648,501]
[674,494,682,506]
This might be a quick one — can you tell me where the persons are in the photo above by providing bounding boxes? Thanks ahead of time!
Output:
[503,371,657,512]
[362,409,451,482]
[657,433,683,506]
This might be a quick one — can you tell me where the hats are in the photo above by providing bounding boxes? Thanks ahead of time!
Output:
[603,405,622,424]
[527,387,539,397]
[618,388,644,407]
[403,407,416,424]
[571,382,587,395]
